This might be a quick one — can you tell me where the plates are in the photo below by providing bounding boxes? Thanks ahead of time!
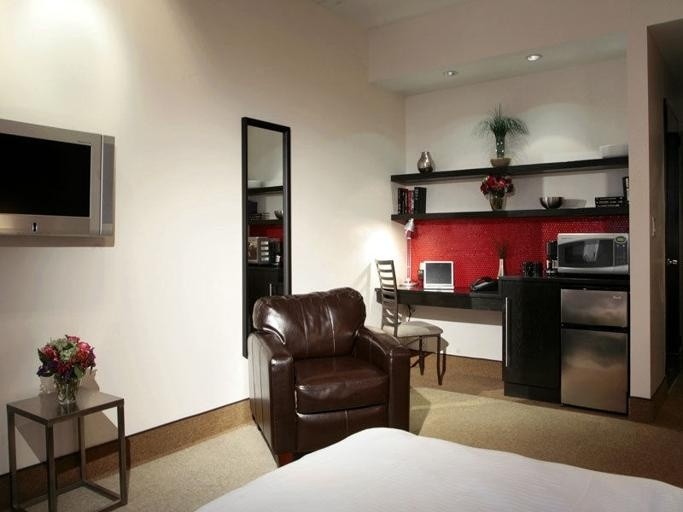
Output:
[398,283,417,287]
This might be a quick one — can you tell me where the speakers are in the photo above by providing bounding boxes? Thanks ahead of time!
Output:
[102,134,114,233]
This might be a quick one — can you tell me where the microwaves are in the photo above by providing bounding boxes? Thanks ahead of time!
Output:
[246,237,266,265]
[557,232,629,273]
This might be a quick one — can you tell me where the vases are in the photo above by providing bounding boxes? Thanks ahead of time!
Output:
[489,192,506,210]
[53,378,80,406]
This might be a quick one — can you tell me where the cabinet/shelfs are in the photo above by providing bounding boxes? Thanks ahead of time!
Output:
[375,156,629,311]
[499,277,560,403]
[248,187,284,304]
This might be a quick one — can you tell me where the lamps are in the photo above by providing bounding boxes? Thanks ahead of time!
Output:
[398,218,419,288]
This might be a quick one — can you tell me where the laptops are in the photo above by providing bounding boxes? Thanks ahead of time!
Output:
[423,260,455,294]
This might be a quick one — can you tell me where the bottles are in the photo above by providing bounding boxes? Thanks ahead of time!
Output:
[417,151,434,172]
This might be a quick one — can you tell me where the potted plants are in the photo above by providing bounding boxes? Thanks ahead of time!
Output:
[476,103,529,167]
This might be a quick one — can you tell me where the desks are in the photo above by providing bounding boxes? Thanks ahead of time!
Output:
[6,386,128,512]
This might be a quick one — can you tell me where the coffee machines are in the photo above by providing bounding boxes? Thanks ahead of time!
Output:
[545,240,557,276]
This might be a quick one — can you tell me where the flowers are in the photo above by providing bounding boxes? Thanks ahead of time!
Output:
[480,174,514,195]
[35,333,97,382]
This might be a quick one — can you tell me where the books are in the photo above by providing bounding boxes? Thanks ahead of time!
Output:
[398,187,426,215]
[595,197,629,208]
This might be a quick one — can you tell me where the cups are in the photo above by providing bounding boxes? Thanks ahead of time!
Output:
[248,180,260,188]
[599,144,627,158]
[520,261,544,278]
[417,270,423,280]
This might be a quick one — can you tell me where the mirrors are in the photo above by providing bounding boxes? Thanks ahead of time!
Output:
[241,116,292,359]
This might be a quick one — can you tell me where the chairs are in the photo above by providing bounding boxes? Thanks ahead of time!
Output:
[374,258,443,385]
[247,287,411,468]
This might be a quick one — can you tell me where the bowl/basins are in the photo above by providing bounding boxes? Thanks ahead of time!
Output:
[274,210,282,220]
[539,196,562,208]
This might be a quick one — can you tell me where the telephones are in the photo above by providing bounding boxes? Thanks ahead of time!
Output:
[470,277,497,292]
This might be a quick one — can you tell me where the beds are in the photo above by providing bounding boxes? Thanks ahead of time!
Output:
[194,427,683,512]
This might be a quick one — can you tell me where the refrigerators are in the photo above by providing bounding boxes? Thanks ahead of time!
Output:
[559,287,628,416]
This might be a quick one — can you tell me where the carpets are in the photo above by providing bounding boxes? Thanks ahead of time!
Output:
[26,386,683,512]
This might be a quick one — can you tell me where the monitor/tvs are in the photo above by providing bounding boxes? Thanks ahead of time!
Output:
[0,119,101,236]
